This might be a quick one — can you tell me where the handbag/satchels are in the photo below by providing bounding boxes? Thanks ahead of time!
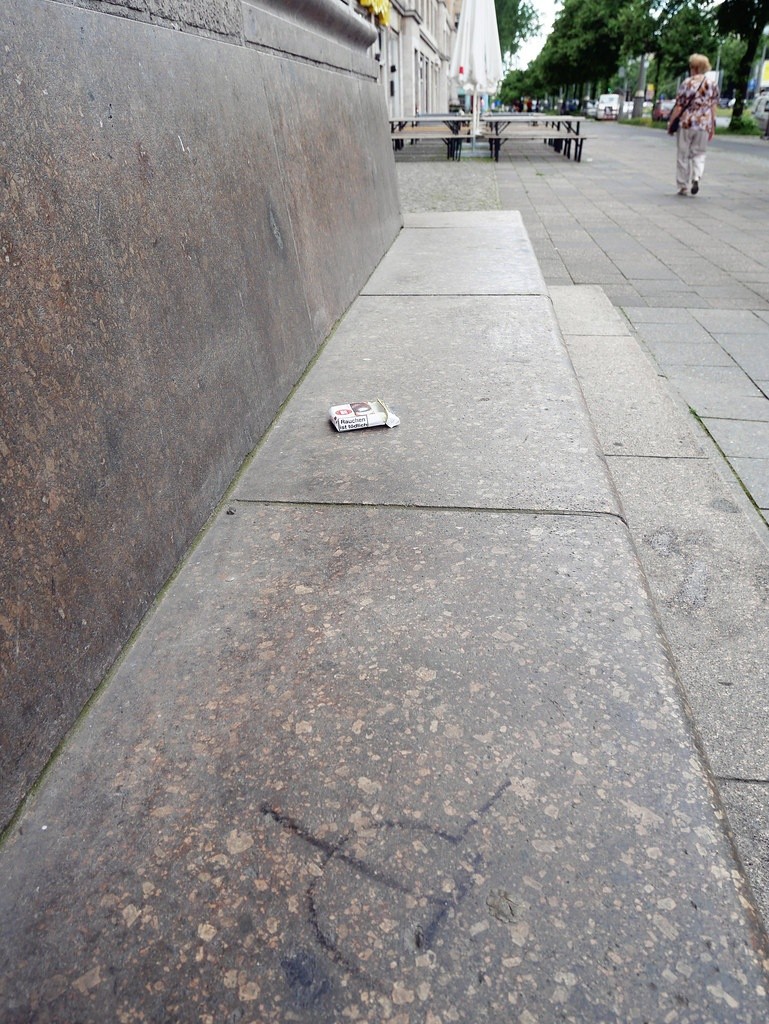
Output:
[665,115,680,135]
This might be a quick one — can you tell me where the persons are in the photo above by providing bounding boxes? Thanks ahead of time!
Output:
[527,98,533,112]
[667,53,720,195]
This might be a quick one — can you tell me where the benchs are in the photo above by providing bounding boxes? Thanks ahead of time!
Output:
[481,127,598,162]
[388,132,474,162]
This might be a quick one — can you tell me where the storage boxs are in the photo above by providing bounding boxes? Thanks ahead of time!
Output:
[328,398,401,432]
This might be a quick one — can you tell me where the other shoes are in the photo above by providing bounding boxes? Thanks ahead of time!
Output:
[678,189,687,195]
[691,179,699,194]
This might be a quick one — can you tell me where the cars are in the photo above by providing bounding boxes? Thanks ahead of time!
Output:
[752,91,769,131]
[652,100,675,122]
[531,93,653,120]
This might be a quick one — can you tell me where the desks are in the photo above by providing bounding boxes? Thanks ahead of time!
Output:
[482,111,586,162]
[389,113,474,163]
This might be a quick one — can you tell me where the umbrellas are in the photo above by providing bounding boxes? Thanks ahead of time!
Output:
[448,0,506,152]
[361,0,390,60]
